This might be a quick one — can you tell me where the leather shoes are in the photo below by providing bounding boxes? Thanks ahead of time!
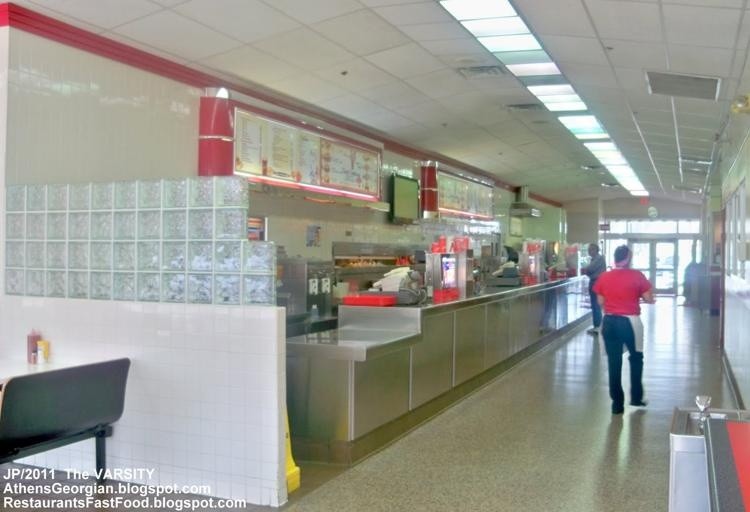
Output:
[588,328,599,333]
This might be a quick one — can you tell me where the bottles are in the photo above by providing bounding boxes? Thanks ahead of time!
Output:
[27,327,41,364]
[309,304,320,318]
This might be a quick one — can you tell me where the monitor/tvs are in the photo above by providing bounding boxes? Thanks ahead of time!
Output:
[391,174,420,225]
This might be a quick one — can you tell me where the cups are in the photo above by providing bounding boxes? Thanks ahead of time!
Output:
[431,242,439,252]
[439,235,469,252]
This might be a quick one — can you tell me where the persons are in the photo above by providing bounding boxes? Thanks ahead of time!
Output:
[579,242,607,336]
[589,244,656,415]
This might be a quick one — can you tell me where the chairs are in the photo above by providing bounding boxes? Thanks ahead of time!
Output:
[2,355,132,491]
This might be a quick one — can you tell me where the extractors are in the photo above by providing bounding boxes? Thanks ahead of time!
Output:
[509,183,543,219]
